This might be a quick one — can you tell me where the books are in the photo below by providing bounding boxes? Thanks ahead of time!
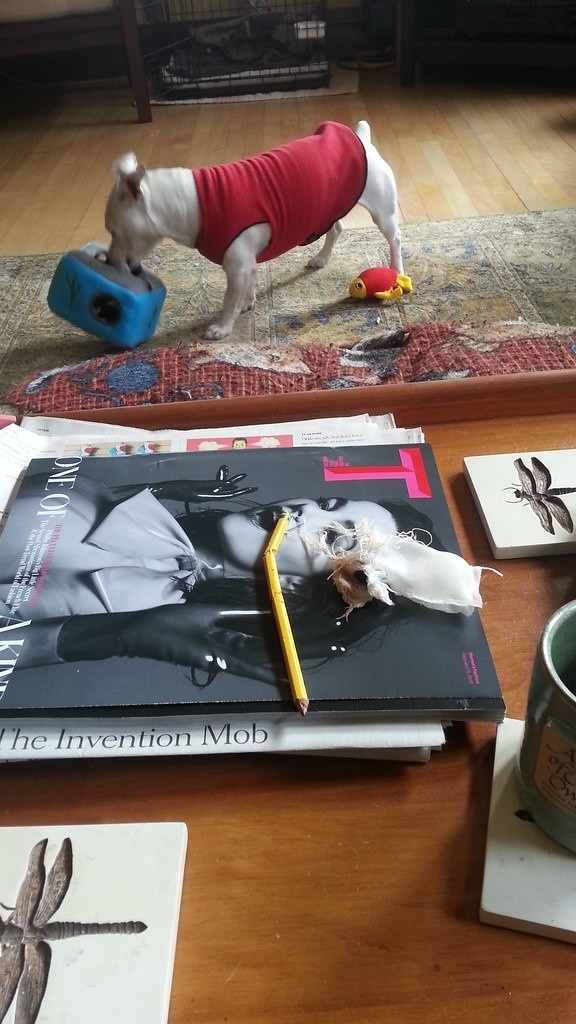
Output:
[0,416,506,762]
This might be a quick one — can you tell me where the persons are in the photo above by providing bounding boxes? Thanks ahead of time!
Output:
[0,466,445,686]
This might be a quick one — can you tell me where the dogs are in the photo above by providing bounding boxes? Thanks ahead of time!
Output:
[104,117,405,342]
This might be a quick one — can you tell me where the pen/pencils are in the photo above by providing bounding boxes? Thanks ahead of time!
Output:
[262,510,312,718]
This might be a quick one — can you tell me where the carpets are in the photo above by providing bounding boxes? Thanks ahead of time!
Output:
[1,206,574,416]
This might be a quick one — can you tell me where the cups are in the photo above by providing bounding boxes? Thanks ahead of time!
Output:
[519,595,576,857]
